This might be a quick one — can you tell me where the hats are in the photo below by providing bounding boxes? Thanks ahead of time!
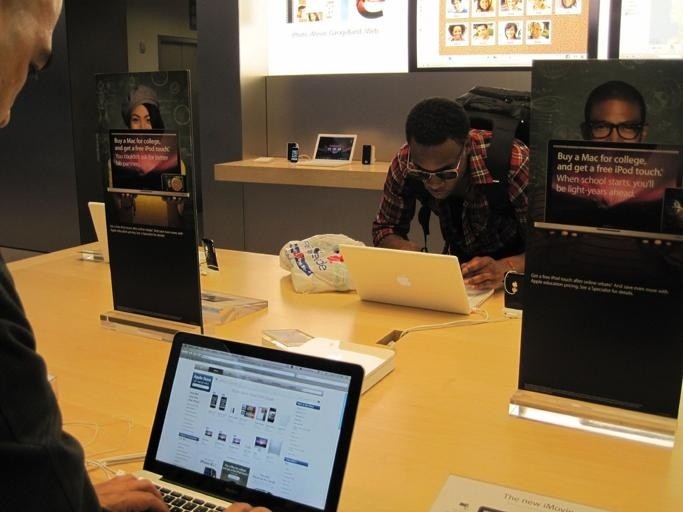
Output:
[122,85,158,124]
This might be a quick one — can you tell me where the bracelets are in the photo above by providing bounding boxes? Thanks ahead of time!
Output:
[505,256,515,271]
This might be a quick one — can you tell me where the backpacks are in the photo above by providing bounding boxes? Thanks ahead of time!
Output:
[410,83,531,255]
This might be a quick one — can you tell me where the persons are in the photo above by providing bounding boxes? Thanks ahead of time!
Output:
[104,82,187,225]
[0,1,272,512]
[307,12,319,22]
[446,1,576,43]
[372,98,532,290]
[296,5,305,18]
[578,78,648,143]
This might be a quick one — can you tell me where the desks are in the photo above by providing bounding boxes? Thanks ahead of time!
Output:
[4,241,683,512]
[212,155,395,192]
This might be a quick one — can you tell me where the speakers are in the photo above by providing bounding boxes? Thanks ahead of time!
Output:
[362,144,375,165]
[288,142,299,159]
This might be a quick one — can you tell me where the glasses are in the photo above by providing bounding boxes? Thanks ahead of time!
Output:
[582,120,642,141]
[405,145,463,182]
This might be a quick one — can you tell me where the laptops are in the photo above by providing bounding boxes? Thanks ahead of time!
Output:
[338,243,495,315]
[296,134,357,167]
[84,332,364,512]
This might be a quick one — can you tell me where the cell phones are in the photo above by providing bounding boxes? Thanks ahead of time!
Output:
[504,272,525,311]
[291,147,298,163]
